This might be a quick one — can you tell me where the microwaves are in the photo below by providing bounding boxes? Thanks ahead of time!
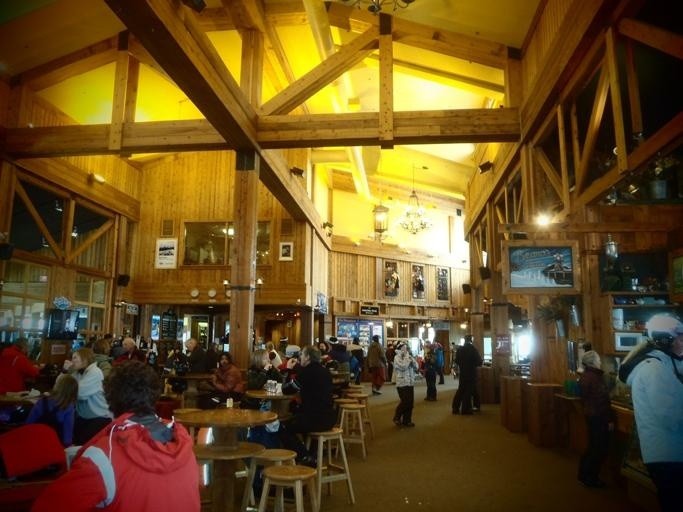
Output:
[613,332,643,351]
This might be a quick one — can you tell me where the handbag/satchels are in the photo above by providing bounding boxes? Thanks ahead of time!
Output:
[196,379,216,392]
[167,377,188,393]
[350,355,359,370]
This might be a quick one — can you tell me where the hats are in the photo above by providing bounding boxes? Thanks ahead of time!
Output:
[328,337,339,347]
[397,341,405,349]
[285,345,301,358]
[647,313,682,349]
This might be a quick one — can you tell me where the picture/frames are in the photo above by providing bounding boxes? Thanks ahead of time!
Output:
[276,239,295,262]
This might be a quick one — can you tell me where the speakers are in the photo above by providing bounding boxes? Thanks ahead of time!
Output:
[478,266,490,281]
[462,284,471,295]
[117,274,130,286]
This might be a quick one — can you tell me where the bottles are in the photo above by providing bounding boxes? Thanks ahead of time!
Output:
[266,379,277,393]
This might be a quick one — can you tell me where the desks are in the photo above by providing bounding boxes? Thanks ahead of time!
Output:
[1,391,49,406]
[167,374,215,408]
[174,408,278,510]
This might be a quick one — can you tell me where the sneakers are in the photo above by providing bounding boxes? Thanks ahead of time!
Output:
[453,407,479,416]
[394,418,401,426]
[403,422,414,426]
[577,475,605,489]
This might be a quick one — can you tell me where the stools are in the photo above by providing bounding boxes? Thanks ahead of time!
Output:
[240,388,371,512]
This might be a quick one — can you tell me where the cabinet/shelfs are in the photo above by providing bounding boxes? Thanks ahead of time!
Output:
[599,289,676,357]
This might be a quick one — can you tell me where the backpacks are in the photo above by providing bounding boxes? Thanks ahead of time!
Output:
[36,396,64,443]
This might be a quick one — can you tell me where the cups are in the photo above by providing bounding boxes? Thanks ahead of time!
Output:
[613,308,624,331]
[631,278,638,290]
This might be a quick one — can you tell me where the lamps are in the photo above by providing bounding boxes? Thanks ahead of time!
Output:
[478,161,493,174]
[370,190,388,242]
[400,161,430,235]
[290,166,304,180]
